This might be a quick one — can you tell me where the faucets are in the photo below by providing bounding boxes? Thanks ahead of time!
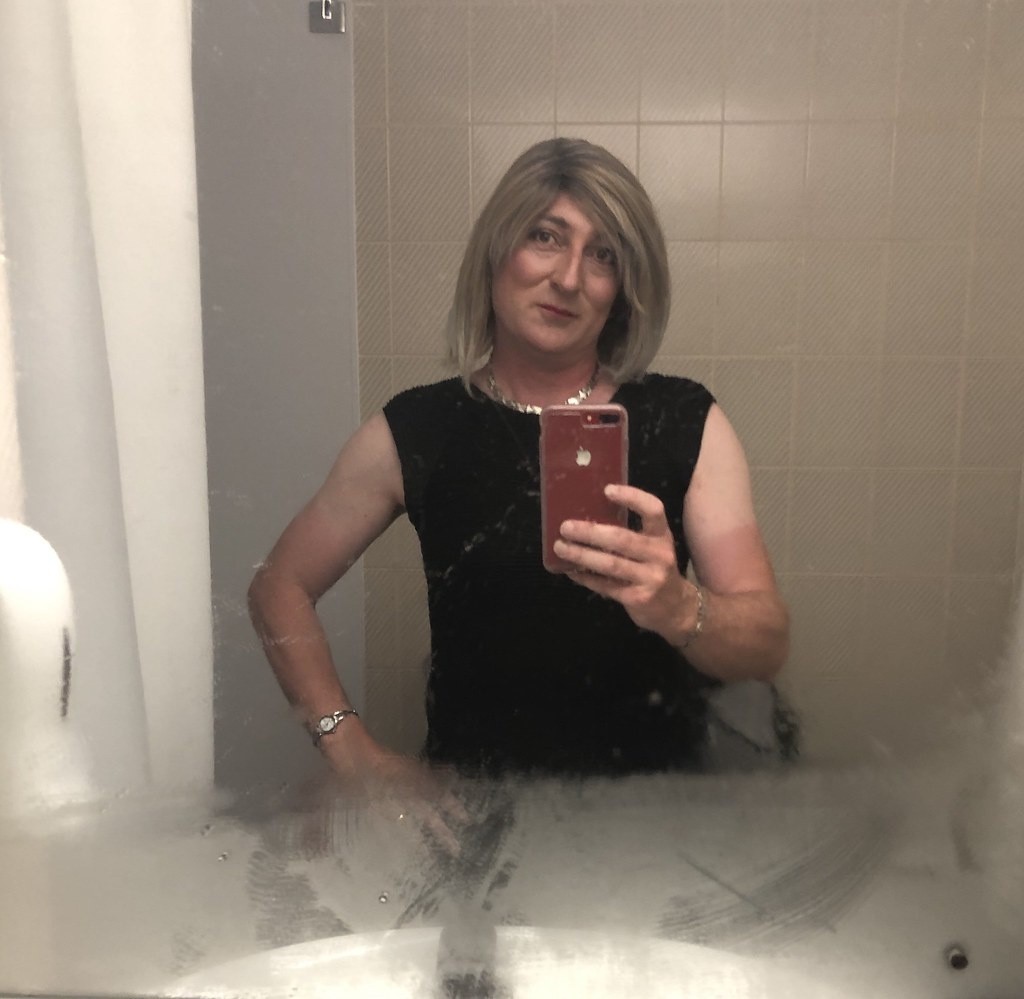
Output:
[431,772,528,999]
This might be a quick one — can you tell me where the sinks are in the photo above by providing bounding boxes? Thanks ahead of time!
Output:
[161,923,845,999]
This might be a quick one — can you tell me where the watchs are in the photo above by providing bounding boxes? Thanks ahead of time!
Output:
[312,708,359,745]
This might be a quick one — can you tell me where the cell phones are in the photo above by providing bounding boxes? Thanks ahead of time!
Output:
[539,403,630,572]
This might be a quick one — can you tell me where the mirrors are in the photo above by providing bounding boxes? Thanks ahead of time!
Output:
[0,0,1024,999]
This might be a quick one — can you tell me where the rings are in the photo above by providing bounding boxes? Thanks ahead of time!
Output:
[395,813,409,823]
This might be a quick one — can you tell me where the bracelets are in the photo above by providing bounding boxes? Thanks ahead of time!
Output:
[674,580,705,648]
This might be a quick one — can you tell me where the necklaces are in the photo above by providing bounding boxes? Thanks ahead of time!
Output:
[485,352,600,415]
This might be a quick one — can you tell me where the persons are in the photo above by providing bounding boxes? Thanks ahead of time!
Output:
[248,137,788,857]
